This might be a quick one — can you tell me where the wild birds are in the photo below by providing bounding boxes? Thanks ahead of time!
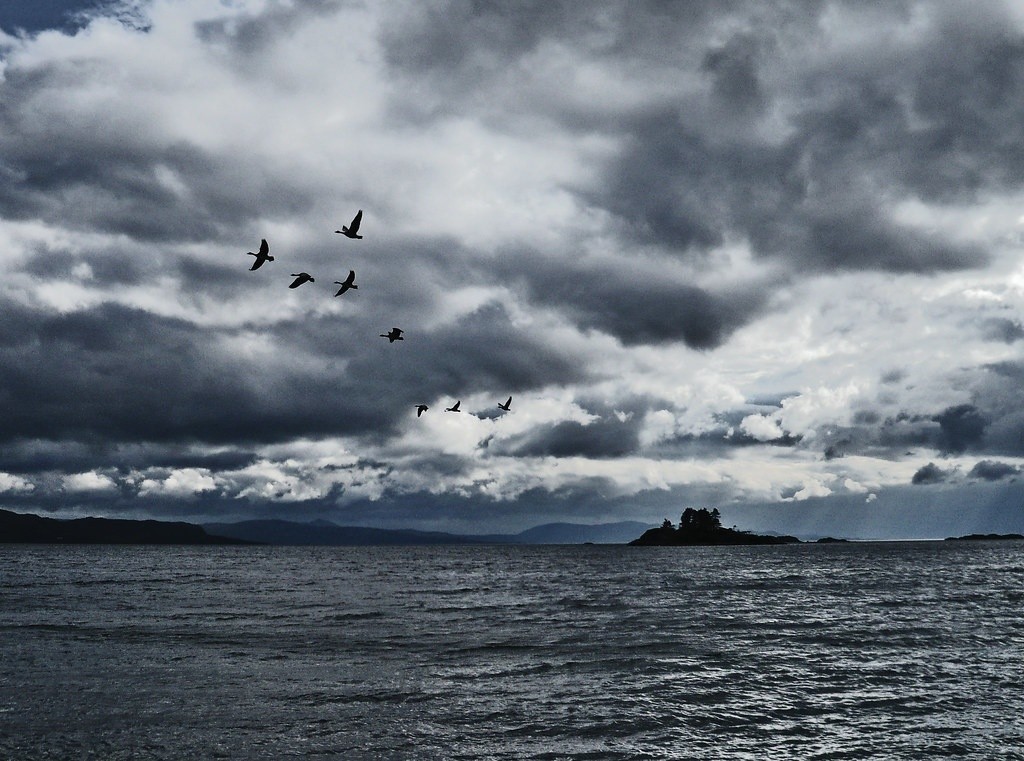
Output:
[289,273,315,289]
[498,396,512,411]
[335,210,363,239]
[380,328,404,343]
[334,270,358,297]
[247,239,274,271]
[444,401,460,412]
[415,405,429,418]
